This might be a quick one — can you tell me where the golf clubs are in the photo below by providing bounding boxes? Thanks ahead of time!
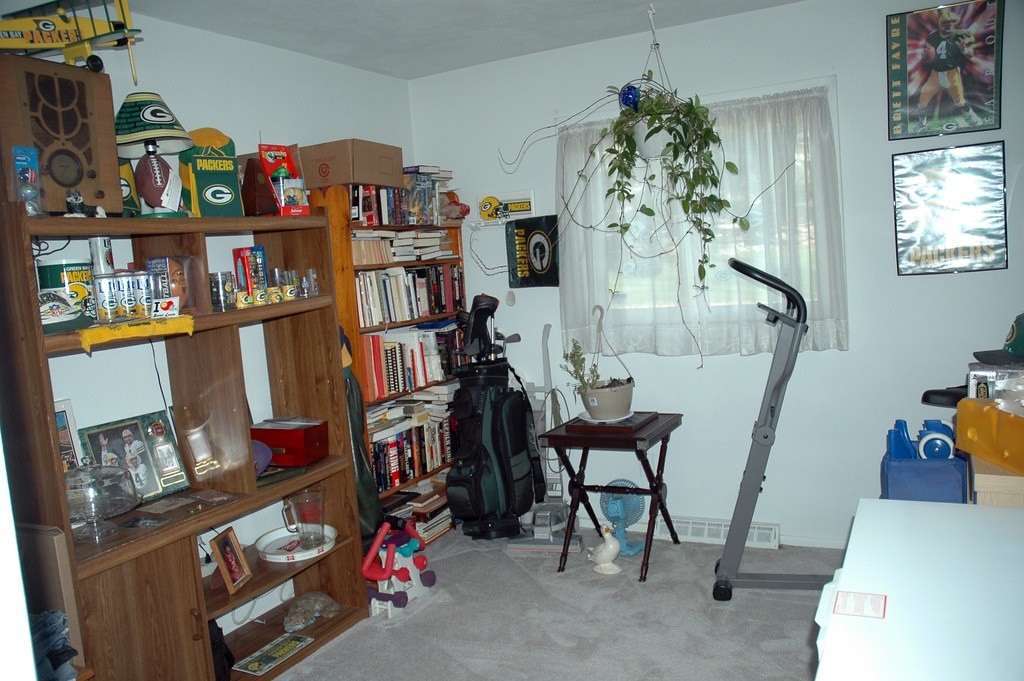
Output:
[494,329,521,360]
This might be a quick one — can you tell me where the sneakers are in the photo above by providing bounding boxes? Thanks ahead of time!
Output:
[913,114,927,132]
[953,103,983,126]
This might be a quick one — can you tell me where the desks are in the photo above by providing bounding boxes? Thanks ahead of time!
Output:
[971,455,1024,509]
[538,413,684,583]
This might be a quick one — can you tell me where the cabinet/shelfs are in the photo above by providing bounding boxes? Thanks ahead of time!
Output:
[310,187,466,546]
[0,200,369,681]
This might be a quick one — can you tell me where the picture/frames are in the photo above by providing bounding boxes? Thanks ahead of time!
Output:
[78,406,190,519]
[209,526,253,595]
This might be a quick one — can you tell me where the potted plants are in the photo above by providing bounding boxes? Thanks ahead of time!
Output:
[560,336,635,419]
[468,73,795,370]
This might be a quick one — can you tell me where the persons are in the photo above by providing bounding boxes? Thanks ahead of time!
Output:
[224,544,246,584]
[121,429,150,465]
[98,432,120,469]
[123,451,155,495]
[913,12,984,134]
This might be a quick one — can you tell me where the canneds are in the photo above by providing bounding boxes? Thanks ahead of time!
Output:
[88,235,153,319]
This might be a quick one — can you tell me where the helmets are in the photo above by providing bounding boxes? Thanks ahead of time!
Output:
[106,453,118,461]
[126,452,141,466]
[939,12,958,33]
[122,429,133,439]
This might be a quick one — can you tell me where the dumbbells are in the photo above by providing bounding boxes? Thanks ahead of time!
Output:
[365,533,437,610]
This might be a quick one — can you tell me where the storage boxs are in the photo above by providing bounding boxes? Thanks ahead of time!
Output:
[238,139,405,189]
[880,440,967,503]
[251,421,331,467]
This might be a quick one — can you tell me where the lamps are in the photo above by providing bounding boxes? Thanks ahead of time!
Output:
[114,92,194,219]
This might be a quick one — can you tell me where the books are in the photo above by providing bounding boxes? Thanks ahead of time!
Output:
[348,164,467,545]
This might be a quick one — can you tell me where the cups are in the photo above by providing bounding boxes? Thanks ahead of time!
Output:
[282,485,325,548]
[281,285,295,301]
[285,270,300,297]
[267,287,280,303]
[251,290,266,305]
[301,269,318,295]
[234,291,248,308]
[209,272,236,311]
[266,268,283,286]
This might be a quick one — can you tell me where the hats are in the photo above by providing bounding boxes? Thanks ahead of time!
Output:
[973,313,1024,365]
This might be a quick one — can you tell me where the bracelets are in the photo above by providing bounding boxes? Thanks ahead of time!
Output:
[961,47,969,55]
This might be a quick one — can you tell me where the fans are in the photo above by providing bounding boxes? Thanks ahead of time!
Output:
[599,478,645,557]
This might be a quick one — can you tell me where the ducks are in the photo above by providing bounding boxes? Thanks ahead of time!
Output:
[586,524,623,572]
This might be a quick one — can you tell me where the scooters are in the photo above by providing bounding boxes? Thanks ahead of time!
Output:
[712,257,834,601]
